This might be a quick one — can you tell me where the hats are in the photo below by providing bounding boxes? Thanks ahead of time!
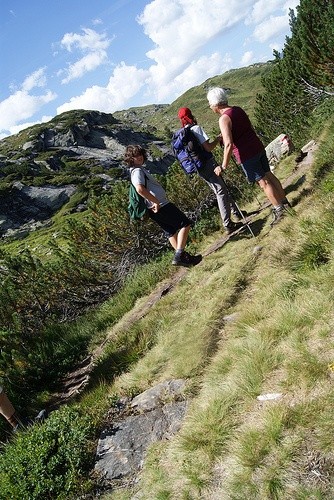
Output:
[178,107,193,128]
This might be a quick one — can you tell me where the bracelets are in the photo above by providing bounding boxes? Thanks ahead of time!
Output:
[219,163,225,173]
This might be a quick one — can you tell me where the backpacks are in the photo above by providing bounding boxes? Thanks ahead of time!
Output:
[128,167,150,224]
[171,123,213,175]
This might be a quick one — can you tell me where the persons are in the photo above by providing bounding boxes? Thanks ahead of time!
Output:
[207,87,293,225]
[178,108,247,235]
[124,145,202,268]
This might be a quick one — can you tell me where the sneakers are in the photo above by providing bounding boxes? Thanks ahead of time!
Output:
[225,221,243,235]
[172,251,202,267]
[234,210,247,221]
[270,199,292,225]
